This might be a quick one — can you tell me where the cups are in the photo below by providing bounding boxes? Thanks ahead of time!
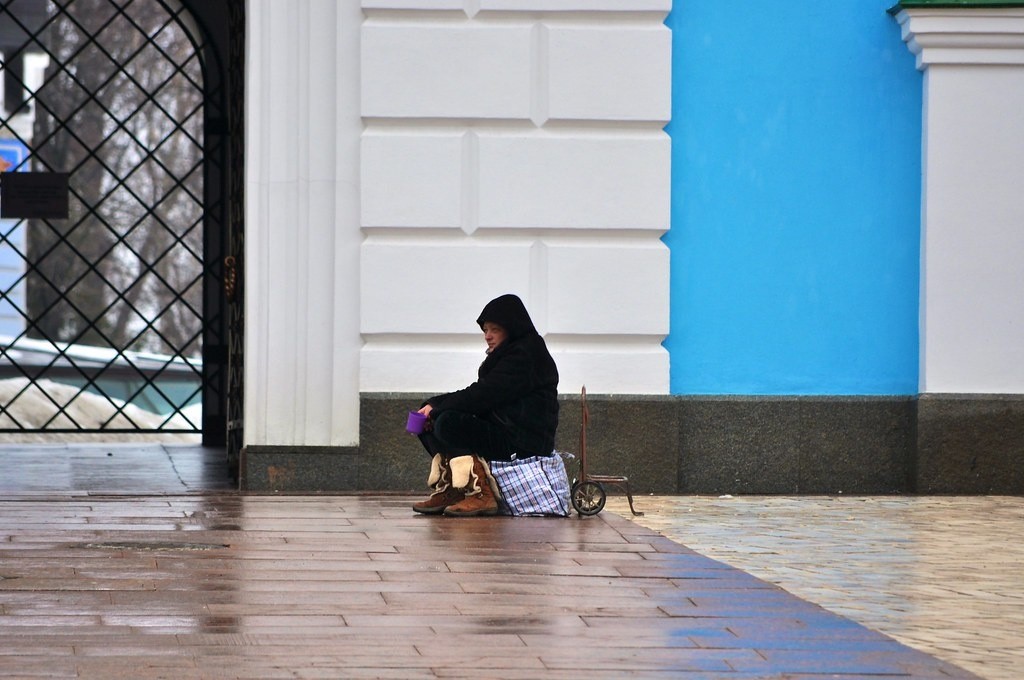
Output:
[406,412,427,434]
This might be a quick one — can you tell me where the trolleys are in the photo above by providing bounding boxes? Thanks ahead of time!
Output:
[571,385,645,518]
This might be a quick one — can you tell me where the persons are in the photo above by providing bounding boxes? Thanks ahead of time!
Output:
[412,292,560,517]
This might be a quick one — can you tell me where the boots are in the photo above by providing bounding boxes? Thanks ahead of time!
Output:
[412,453,464,515]
[443,455,498,518]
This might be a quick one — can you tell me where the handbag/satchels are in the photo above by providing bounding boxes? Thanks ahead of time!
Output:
[490,450,571,517]
